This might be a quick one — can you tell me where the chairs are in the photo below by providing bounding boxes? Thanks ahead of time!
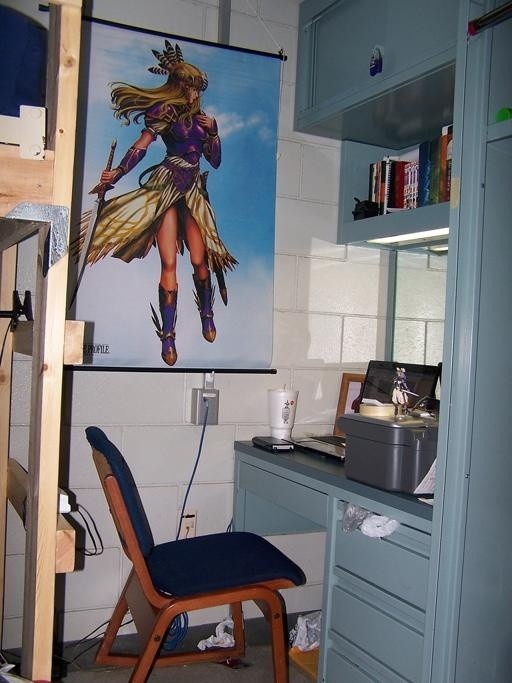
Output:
[85,425,309,683]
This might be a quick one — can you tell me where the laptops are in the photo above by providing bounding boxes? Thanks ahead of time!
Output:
[282,360,439,461]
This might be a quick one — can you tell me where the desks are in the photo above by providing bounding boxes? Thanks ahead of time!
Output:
[230,440,435,681]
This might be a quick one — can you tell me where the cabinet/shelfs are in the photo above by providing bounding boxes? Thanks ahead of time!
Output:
[290,0,461,252]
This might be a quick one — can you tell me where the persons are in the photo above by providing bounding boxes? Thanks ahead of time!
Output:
[69,39,223,366]
[390,366,411,416]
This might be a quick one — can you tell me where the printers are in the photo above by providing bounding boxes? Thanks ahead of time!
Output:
[339,407,439,497]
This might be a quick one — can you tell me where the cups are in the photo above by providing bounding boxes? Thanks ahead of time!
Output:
[268,388,298,438]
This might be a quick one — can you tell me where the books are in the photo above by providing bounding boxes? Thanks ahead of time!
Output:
[368,124,453,215]
